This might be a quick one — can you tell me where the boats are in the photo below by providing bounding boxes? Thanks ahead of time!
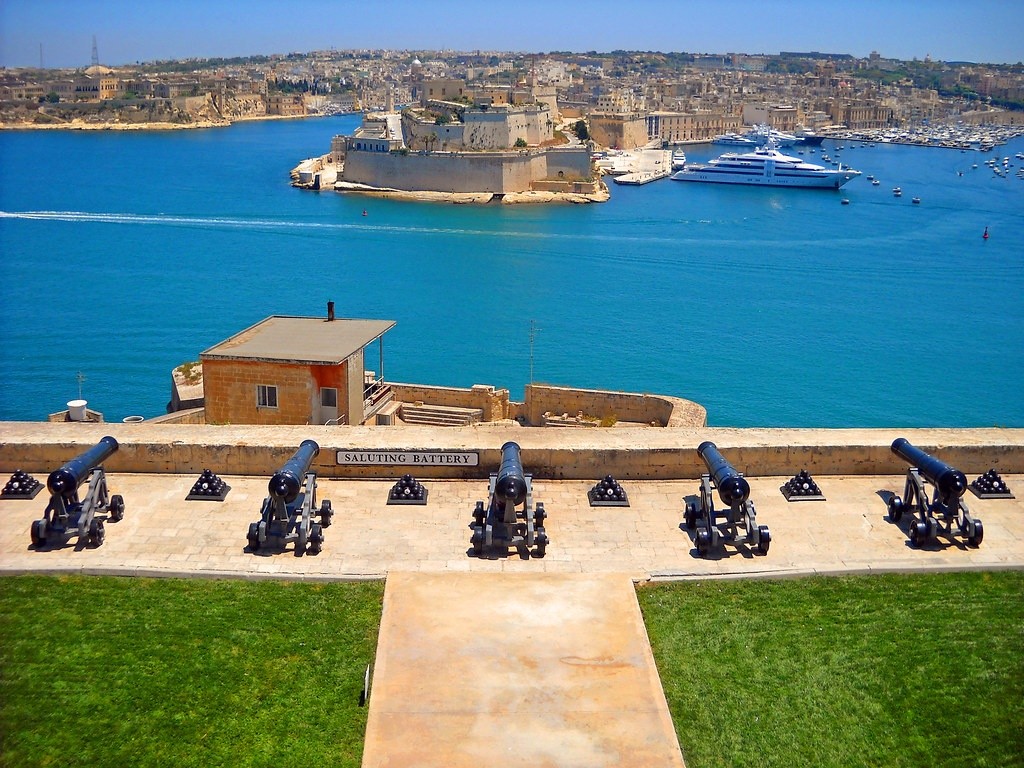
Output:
[671,147,686,174]
[788,127,828,144]
[670,124,863,188]
[745,126,808,148]
[710,130,757,146]
